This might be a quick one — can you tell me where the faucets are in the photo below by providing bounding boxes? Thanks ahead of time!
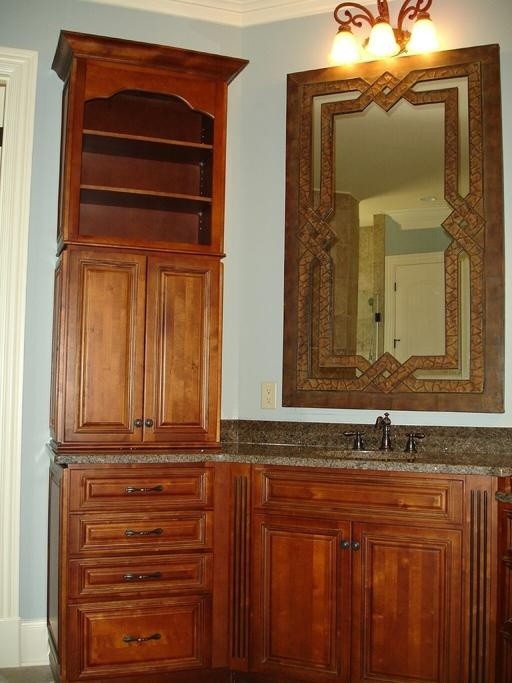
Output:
[376,412,393,450]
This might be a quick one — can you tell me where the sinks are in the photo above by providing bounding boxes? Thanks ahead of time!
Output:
[317,450,424,461]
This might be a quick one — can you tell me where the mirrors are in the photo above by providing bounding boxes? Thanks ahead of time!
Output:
[280,42,507,414]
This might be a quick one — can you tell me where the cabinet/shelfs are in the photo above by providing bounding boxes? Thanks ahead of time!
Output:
[48,249,225,455]
[47,464,216,683]
[494,476,512,683]
[218,465,495,683]
[51,28,250,256]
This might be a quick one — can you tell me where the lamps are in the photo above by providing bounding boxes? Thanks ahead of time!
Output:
[327,1,441,65]
